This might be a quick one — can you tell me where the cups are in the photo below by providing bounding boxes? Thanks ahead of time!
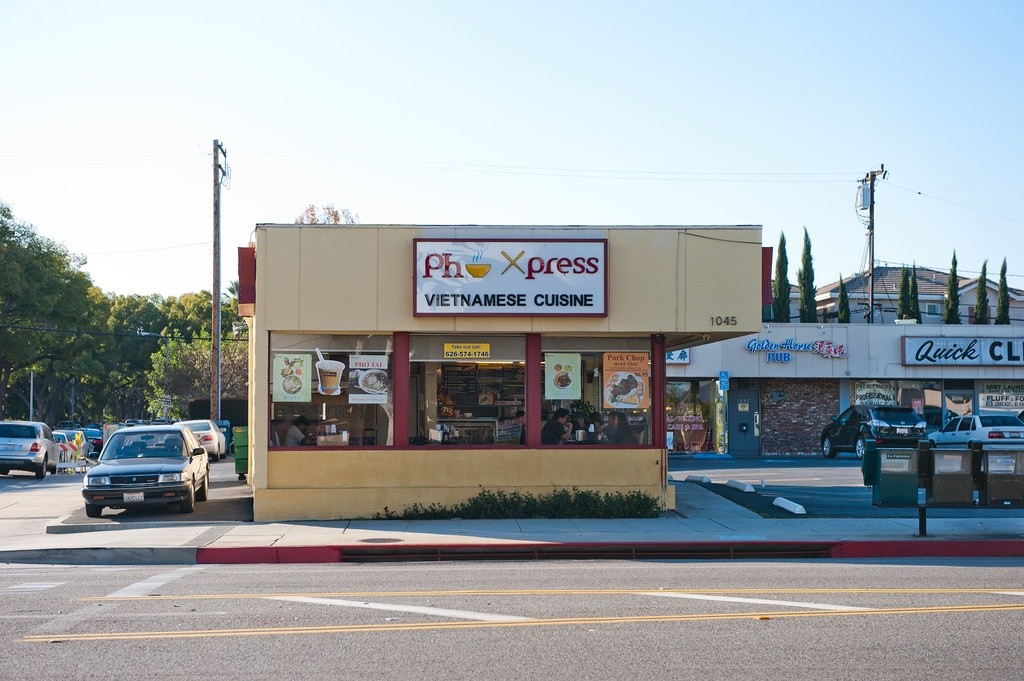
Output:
[315,360,345,395]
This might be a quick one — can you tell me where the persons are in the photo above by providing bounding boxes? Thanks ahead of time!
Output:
[577,412,604,434]
[508,410,525,445]
[595,411,615,436]
[284,416,313,446]
[605,413,636,444]
[540,408,572,444]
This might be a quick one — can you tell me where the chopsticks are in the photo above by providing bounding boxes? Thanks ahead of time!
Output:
[354,385,388,394]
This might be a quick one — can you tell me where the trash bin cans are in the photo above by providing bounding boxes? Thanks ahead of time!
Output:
[925,446,974,506]
[978,443,1024,508]
[872,447,920,508]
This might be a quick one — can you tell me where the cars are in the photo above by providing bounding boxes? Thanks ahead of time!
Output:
[51,420,227,519]
[926,416,1024,451]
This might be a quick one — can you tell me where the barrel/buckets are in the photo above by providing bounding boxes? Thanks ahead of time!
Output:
[576,429,585,441]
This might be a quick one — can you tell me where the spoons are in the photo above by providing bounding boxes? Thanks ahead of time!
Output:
[315,348,324,362]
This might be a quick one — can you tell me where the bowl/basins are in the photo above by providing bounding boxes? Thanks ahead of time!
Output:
[359,369,389,394]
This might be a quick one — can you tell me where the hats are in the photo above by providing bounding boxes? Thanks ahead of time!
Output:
[297,416,311,426]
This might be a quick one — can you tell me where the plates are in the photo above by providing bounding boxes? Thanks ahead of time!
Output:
[554,370,572,389]
[554,363,563,372]
[282,374,304,394]
[564,363,574,372]
[604,371,648,408]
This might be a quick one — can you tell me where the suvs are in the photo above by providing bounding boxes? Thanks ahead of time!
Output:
[0,420,59,482]
[822,404,927,461]
